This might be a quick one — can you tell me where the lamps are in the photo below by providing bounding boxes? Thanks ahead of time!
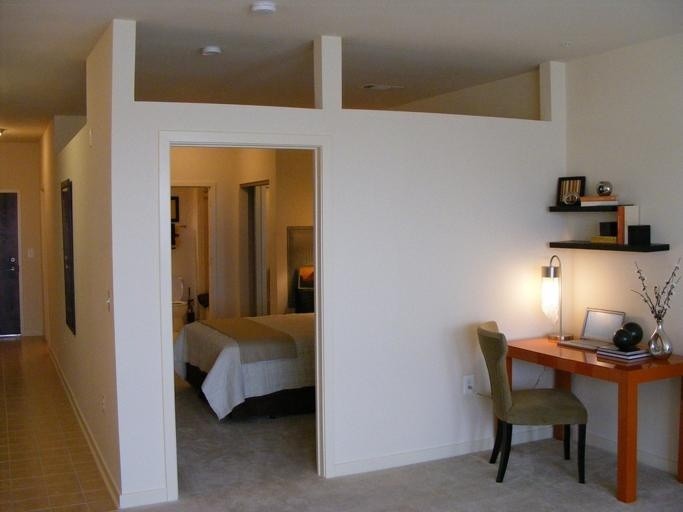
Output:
[541,255,575,341]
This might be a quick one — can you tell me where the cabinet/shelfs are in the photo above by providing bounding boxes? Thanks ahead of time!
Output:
[174,301,188,333]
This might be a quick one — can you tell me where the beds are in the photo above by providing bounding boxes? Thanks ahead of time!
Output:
[179,310,315,423]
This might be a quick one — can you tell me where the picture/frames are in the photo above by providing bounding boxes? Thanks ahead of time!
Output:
[557,176,586,207]
[171,196,180,223]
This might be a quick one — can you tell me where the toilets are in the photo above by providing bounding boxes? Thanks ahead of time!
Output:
[172,276,187,332]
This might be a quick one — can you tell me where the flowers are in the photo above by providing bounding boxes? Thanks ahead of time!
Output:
[631,257,682,320]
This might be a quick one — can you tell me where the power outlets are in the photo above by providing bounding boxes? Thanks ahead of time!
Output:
[462,373,476,397]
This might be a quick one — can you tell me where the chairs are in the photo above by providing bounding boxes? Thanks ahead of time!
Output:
[477,321,588,484]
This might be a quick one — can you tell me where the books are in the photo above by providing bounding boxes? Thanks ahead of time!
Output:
[596,344,653,368]
[579,194,640,246]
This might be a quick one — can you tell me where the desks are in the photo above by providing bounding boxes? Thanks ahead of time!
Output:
[506,334,680,504]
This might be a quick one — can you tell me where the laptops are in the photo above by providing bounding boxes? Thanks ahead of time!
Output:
[557,307,625,352]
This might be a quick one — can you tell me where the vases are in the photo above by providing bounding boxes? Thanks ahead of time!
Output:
[648,321,673,361]
